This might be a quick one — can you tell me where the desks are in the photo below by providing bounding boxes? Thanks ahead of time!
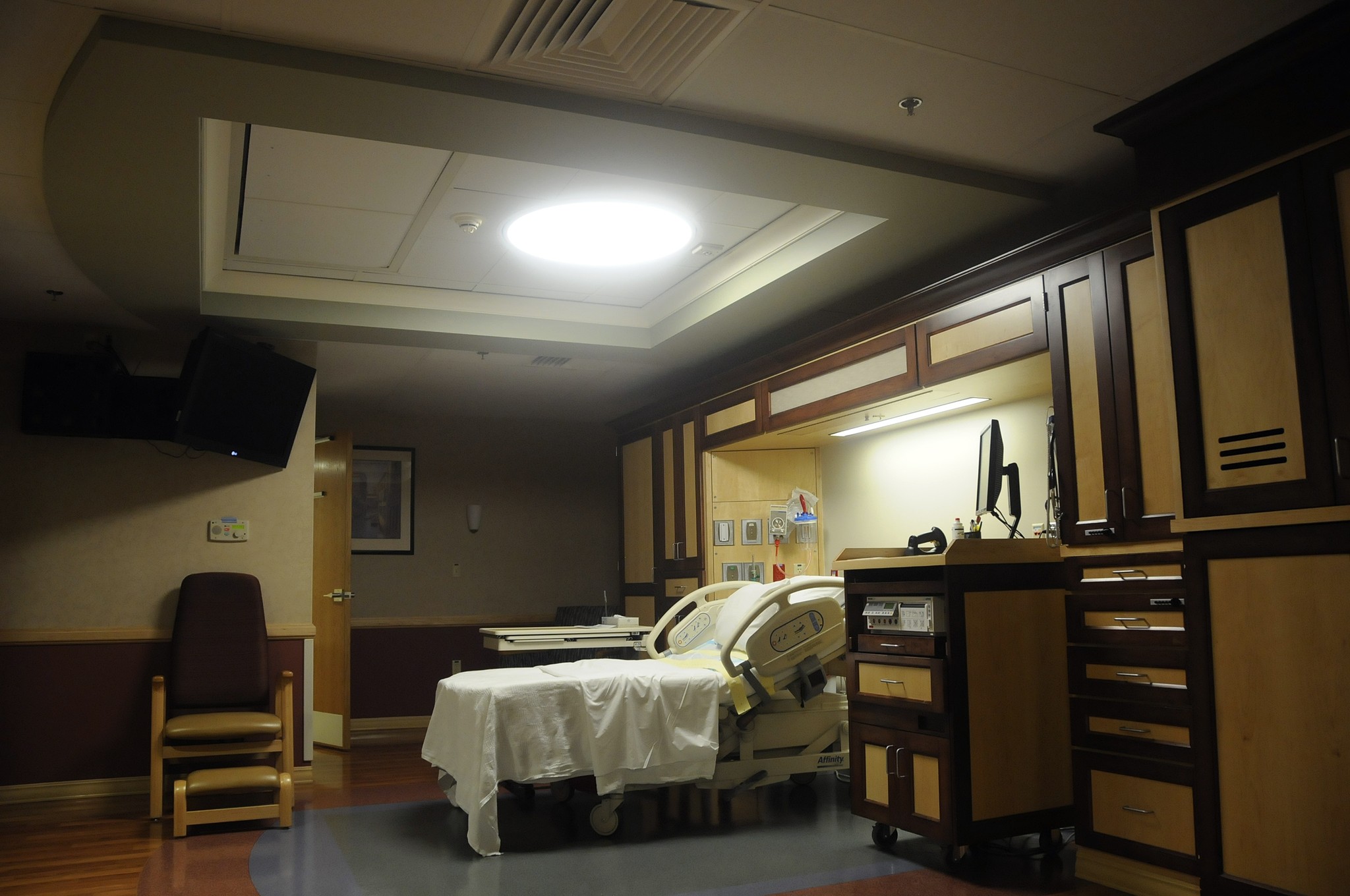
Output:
[474,623,655,669]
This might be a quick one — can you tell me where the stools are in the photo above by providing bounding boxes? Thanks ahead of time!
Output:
[174,766,293,838]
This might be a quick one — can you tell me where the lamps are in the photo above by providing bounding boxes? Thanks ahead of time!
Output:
[466,502,482,534]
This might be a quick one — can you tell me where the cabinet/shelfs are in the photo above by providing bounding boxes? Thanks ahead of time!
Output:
[830,538,1085,876]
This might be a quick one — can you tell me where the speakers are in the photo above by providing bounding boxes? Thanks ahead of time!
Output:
[20,376,179,440]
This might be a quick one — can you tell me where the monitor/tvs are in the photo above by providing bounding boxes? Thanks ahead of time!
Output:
[977,418,1004,517]
[164,332,317,470]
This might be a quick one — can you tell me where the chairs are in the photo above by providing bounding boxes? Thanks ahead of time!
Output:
[150,568,299,820]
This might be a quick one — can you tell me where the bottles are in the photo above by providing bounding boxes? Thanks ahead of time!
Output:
[951,517,965,539]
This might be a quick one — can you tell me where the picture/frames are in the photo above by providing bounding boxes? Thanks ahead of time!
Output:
[349,443,417,558]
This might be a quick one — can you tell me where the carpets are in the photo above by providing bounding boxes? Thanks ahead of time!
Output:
[242,779,931,893]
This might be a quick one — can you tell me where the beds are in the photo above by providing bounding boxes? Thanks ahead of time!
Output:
[423,576,852,856]
